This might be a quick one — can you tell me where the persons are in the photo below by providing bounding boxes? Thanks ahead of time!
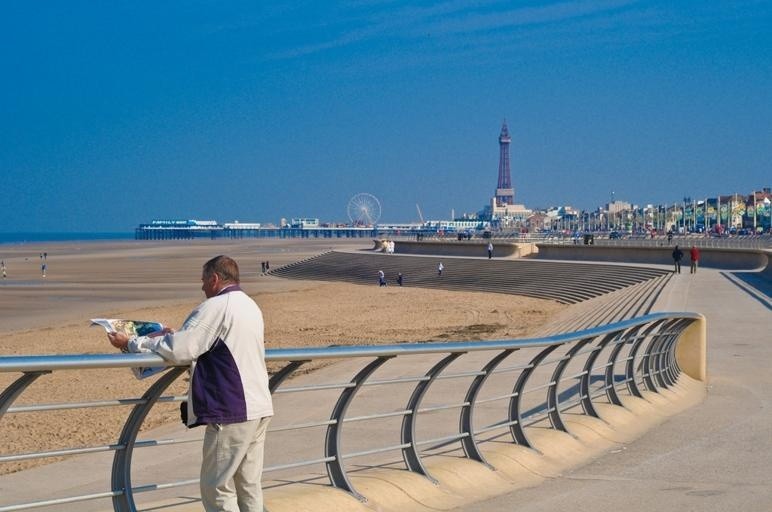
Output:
[666,229,673,245]
[690,245,699,273]
[0,264,7,280]
[378,270,384,284]
[398,272,403,286]
[488,240,494,258]
[672,245,683,273]
[108,256,274,511]
[40,251,47,277]
[438,262,445,275]
